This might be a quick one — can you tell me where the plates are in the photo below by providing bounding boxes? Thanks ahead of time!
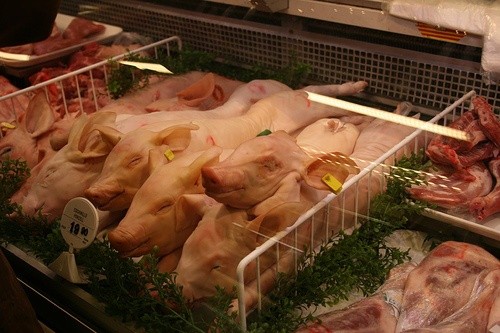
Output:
[0,13,122,67]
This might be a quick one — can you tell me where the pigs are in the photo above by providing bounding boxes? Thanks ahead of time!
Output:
[0,72,370,319]
